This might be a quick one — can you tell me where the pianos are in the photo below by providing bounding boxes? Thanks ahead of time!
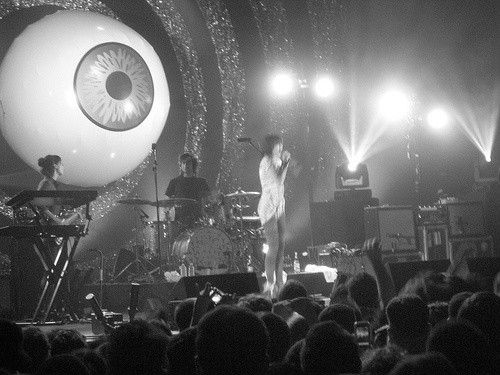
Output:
[0,188,101,326]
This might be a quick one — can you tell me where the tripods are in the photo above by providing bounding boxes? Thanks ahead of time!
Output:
[109,202,155,282]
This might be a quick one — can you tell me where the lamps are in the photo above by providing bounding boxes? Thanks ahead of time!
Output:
[472,151,500,183]
[334,161,369,202]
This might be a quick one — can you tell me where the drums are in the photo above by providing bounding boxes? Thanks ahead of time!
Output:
[233,214,264,239]
[170,224,235,276]
[201,202,236,230]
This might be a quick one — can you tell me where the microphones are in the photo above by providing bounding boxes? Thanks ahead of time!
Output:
[236,137,253,143]
[152,143,157,170]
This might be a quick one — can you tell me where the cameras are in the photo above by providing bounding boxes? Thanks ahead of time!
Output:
[209,286,225,305]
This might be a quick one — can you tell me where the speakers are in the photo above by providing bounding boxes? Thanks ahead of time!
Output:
[166,272,261,329]
[378,259,463,310]
[453,256,500,294]
[364,206,419,254]
[441,200,486,238]
[287,272,328,296]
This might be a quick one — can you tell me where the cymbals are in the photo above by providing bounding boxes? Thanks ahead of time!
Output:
[116,197,153,205]
[223,189,262,199]
[153,197,200,207]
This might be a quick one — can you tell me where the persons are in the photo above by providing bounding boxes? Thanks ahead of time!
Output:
[165,152,210,227]
[0,271,500,375]
[36,155,86,321]
[256,134,293,293]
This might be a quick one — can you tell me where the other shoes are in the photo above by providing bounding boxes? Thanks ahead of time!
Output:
[264,281,283,298]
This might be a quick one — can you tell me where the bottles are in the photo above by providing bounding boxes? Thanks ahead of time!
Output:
[294,259,300,274]
[246,255,253,273]
[180,263,187,277]
[188,263,195,276]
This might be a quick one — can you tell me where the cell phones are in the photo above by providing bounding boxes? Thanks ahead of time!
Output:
[354,321,370,353]
